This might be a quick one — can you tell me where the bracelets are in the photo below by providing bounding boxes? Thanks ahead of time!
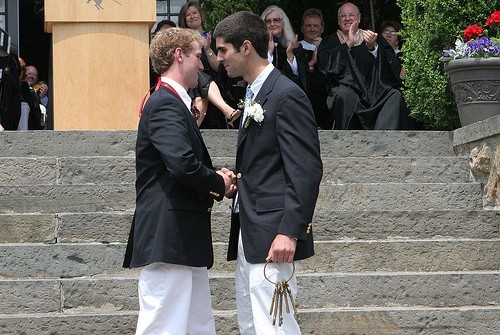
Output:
[288,56,294,62]
[206,50,214,57]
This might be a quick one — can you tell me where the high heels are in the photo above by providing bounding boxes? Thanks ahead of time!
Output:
[226,110,241,128]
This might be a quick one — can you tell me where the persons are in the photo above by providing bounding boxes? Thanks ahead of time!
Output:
[316,3,401,130]
[297,8,334,130]
[261,5,308,96]
[149,20,176,95]
[123,27,237,335]
[0,51,49,131]
[178,2,241,129]
[213,11,323,335]
[380,20,425,130]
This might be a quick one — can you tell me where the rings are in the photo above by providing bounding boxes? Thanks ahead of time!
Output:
[316,43,318,46]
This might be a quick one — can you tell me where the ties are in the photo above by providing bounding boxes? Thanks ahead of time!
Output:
[242,88,254,127]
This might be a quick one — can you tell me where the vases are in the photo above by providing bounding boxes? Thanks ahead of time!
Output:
[444,58,500,126]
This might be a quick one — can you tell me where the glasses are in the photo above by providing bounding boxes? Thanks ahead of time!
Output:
[382,29,396,35]
[266,18,282,23]
[338,13,359,19]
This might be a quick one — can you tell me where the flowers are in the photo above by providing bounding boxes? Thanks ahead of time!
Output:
[192,106,201,119]
[439,11,500,62]
[238,98,264,127]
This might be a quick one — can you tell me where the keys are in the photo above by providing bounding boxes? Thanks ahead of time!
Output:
[269,280,302,326]
